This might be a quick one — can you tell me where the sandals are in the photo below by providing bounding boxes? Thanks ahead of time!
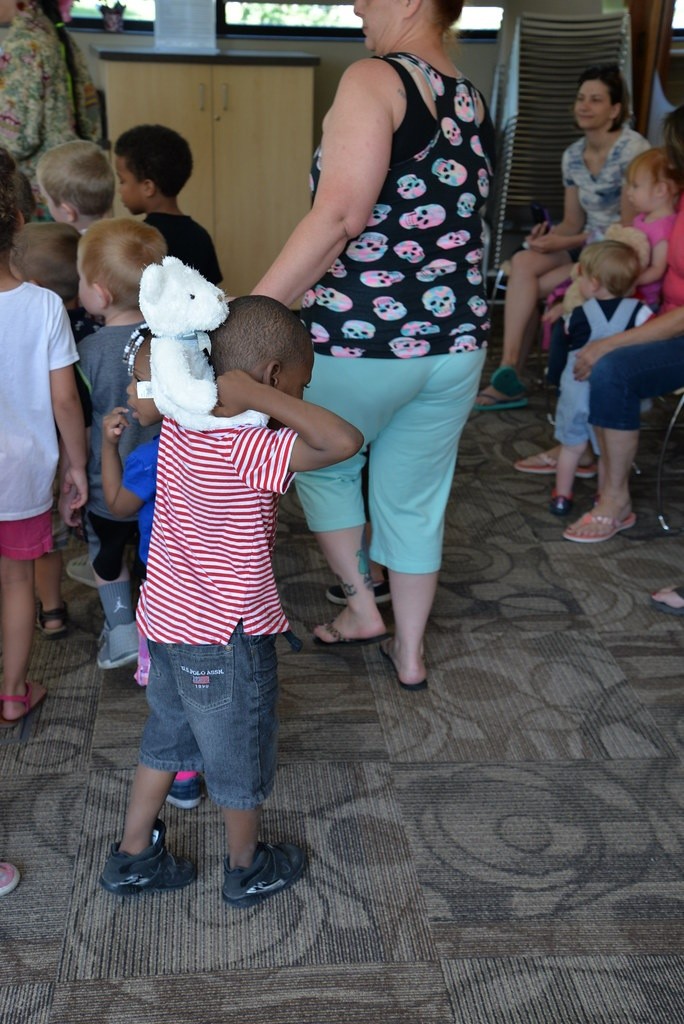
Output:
[0,680,48,728]
[33,600,67,639]
[650,583,684,615]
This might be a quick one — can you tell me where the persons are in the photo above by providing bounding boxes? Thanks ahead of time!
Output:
[98,295,366,908]
[468,60,684,615]
[325,451,390,606]
[0,0,223,892]
[220,0,499,690]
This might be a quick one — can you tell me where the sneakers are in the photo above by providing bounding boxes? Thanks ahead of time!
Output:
[221,840,304,908]
[99,815,197,895]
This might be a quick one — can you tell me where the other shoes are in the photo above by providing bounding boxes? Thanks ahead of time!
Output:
[166,774,204,809]
[97,619,138,669]
[550,483,574,515]
[0,861,20,896]
[66,554,98,589]
[325,581,392,606]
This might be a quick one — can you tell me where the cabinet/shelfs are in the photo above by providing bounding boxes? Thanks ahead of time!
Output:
[91,45,322,309]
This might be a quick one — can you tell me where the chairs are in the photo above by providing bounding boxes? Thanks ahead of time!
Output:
[488,270,684,535]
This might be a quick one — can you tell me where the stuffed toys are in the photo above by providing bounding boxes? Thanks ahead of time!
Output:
[138,257,271,432]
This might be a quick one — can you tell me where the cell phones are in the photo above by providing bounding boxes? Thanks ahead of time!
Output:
[532,202,550,235]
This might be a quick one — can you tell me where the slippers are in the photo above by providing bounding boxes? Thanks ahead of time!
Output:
[563,512,635,542]
[491,366,527,398]
[474,392,528,410]
[514,452,598,478]
[380,636,428,691]
[313,618,393,648]
